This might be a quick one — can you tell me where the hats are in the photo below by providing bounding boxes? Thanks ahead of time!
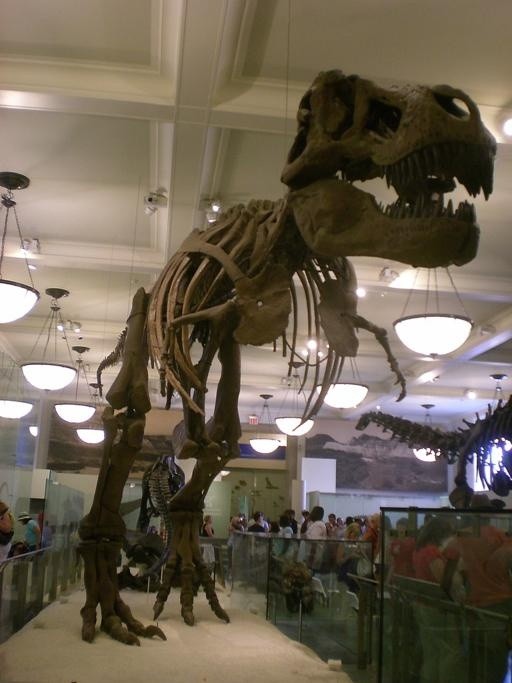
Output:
[17,513,32,521]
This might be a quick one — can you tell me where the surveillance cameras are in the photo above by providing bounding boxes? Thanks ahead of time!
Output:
[142,192,168,216]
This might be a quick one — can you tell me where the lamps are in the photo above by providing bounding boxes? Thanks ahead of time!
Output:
[394,267,474,356]
[249,356,369,454]
[413,405,442,461]
[0,172,106,445]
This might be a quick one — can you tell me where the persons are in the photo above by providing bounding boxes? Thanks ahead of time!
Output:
[202,514,215,538]
[0,501,16,565]
[17,510,42,554]
[40,519,82,569]
[230,506,512,683]
